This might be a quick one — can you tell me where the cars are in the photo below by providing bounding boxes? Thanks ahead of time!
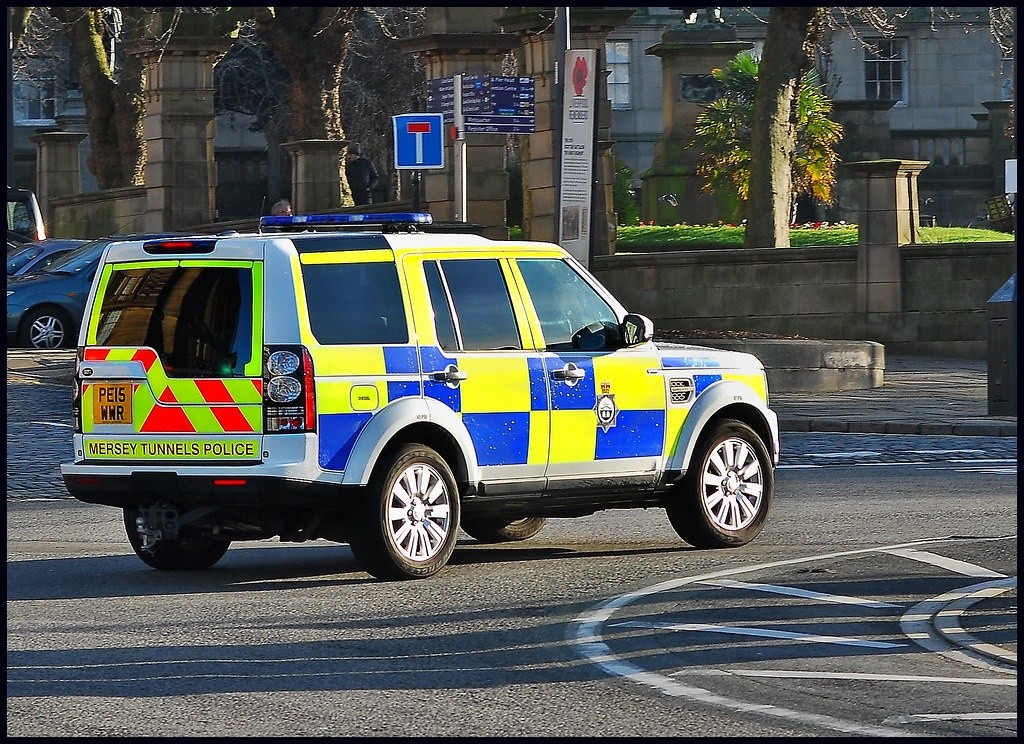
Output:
[7,230,200,347]
[6,185,98,251]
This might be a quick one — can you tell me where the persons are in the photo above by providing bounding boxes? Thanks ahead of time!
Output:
[271,200,291,216]
[344,145,380,206]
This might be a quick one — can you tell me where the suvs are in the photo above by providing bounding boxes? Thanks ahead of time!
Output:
[59,211,780,581]
